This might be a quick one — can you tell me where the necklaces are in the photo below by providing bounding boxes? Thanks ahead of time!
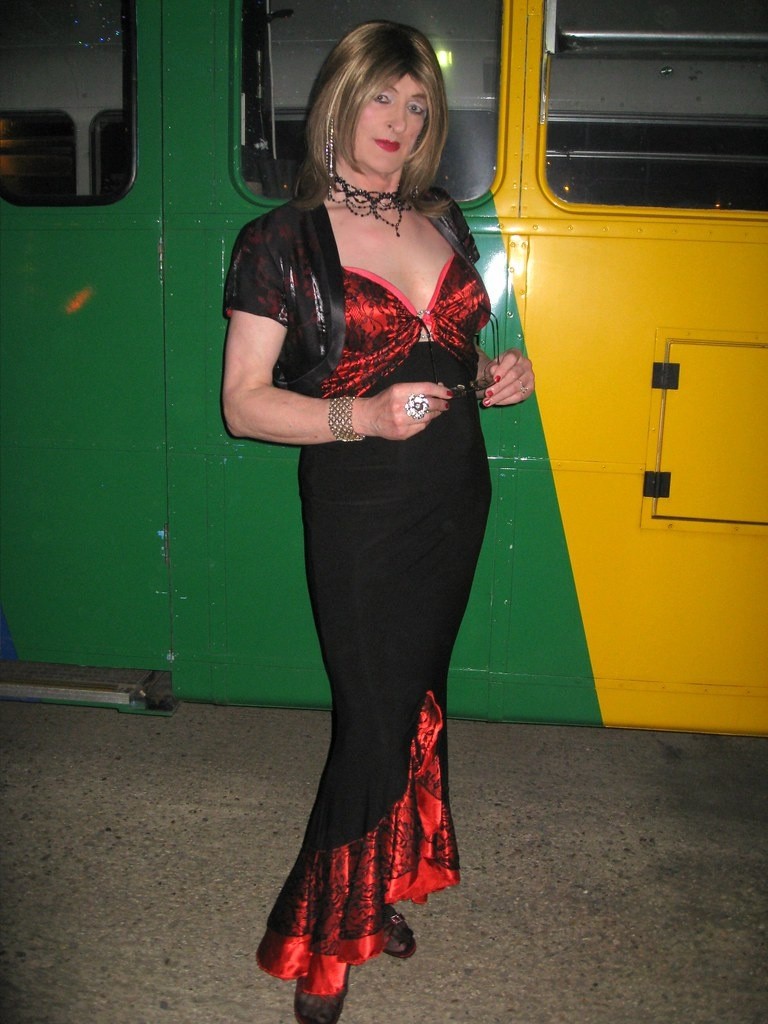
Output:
[329,172,411,235]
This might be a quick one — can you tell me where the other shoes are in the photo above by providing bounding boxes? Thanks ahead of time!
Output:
[376,905,418,959]
[293,952,351,1024]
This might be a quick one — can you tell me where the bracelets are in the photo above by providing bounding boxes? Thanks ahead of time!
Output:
[329,396,366,441]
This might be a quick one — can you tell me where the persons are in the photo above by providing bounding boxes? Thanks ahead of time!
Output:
[220,21,537,1023]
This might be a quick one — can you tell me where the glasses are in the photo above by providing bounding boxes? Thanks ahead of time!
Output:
[416,309,504,400]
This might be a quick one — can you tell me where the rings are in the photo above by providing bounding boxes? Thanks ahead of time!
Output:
[517,379,528,393]
[404,394,429,420]
